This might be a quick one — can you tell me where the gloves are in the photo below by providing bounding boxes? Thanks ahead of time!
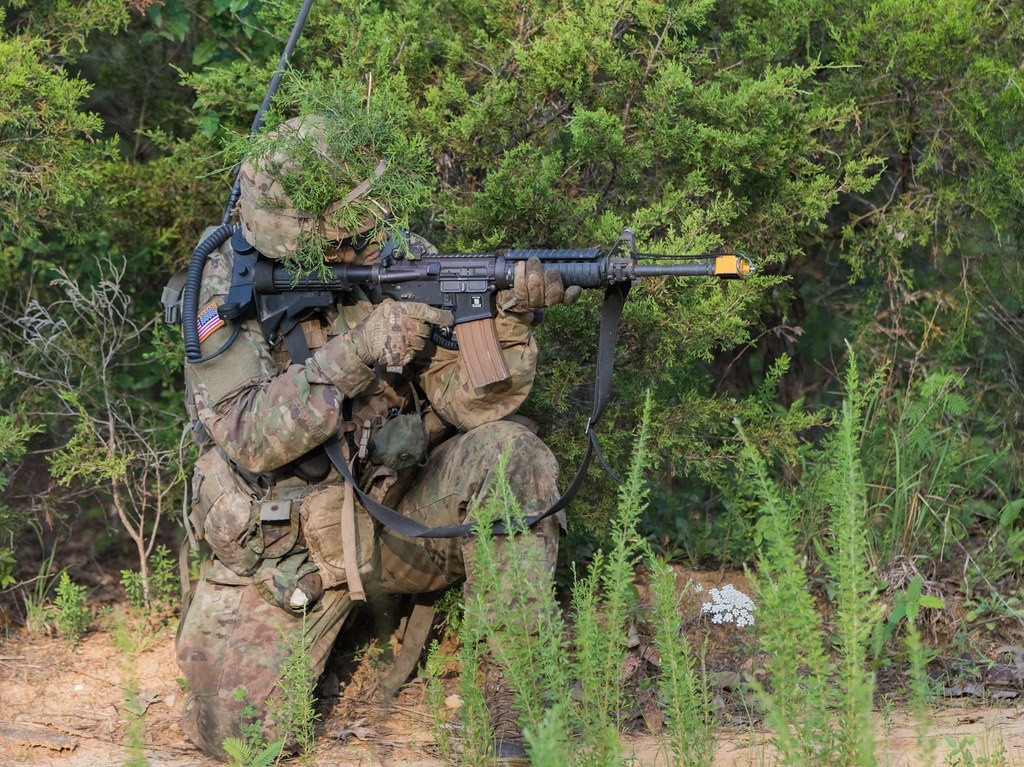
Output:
[347,298,454,374]
[495,256,582,348]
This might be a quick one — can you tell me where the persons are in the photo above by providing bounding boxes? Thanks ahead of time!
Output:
[174,116,578,766]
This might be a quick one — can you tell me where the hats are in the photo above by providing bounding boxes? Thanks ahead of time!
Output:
[236,116,402,259]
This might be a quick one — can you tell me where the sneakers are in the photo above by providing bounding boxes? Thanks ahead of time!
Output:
[480,654,529,767]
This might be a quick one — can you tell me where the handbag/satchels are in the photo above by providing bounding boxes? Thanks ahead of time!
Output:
[188,443,264,577]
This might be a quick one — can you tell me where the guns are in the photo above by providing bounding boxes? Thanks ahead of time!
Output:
[249,223,760,401]
